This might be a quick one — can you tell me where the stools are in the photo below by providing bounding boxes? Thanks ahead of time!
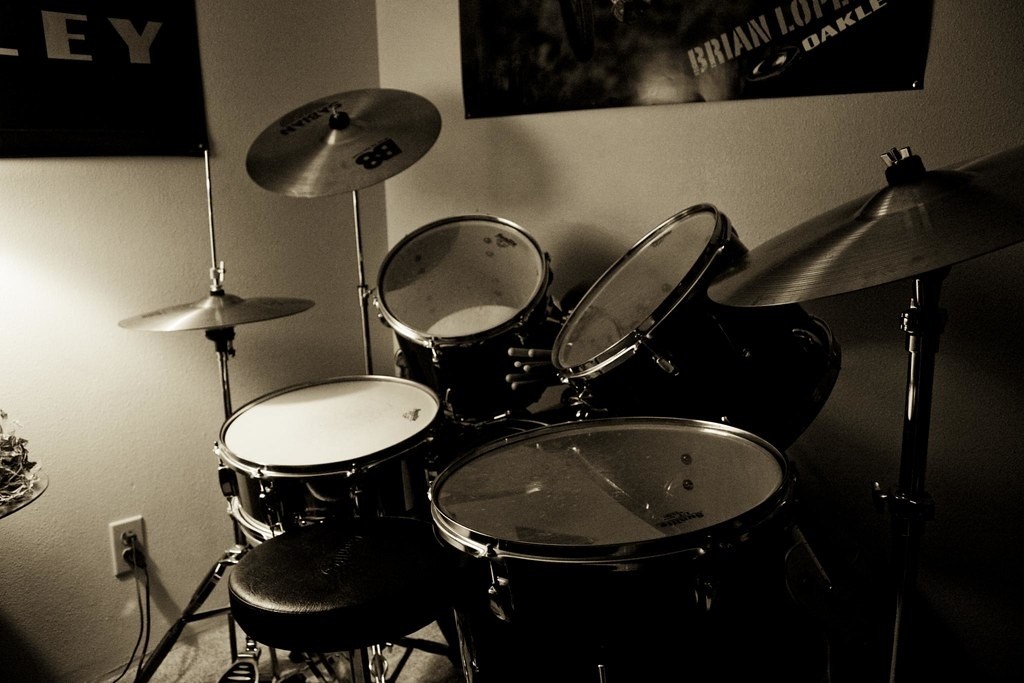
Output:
[227,516,468,683]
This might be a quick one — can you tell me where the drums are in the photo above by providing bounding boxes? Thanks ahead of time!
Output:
[426,414,814,683]
[548,203,844,452]
[374,212,560,432]
[213,372,445,555]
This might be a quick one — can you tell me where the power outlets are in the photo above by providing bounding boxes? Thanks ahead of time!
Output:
[108,515,146,575]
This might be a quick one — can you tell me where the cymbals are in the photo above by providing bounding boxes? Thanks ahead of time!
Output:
[118,291,316,333]
[704,141,1024,311]
[244,85,442,200]
